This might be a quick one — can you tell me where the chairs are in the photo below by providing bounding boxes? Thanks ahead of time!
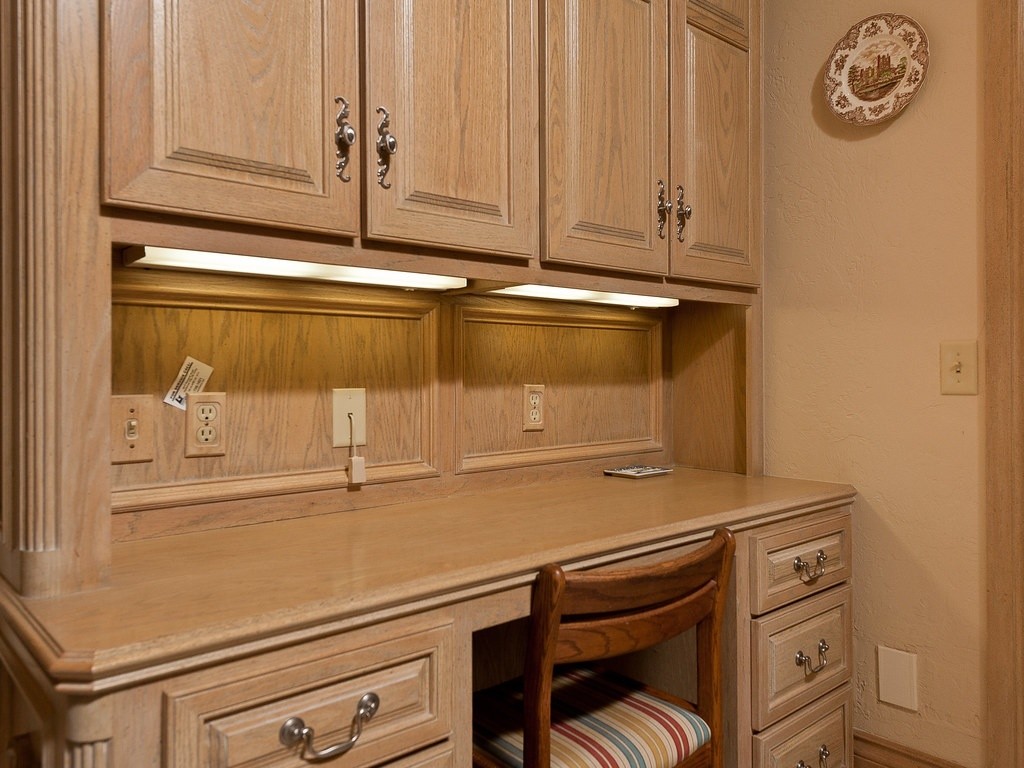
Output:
[473,526,736,768]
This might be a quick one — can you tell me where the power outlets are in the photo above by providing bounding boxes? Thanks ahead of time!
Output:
[183,391,227,456]
[523,384,544,432]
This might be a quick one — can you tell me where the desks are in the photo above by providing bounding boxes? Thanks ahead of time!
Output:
[0,466,857,768]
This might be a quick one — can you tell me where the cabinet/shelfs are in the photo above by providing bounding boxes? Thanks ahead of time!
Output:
[538,0,763,306]
[98,0,541,295]
